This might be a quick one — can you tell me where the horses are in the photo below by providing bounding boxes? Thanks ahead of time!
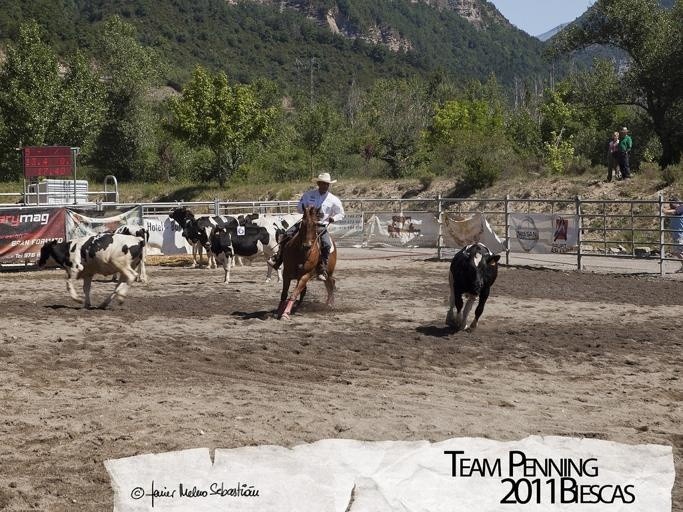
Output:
[275,204,339,323]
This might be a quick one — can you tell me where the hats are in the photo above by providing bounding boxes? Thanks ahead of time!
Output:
[312,173,337,184]
[620,127,630,133]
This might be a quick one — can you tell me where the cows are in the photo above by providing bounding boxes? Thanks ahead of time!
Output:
[36,231,145,310]
[446,241,501,331]
[113,224,149,283]
[167,208,303,283]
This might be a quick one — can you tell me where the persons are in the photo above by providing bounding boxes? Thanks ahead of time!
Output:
[616,126,633,179]
[601,132,621,182]
[263,171,345,283]
[660,196,682,275]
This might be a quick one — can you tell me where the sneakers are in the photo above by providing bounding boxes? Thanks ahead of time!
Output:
[316,274,327,282]
[267,260,280,270]
